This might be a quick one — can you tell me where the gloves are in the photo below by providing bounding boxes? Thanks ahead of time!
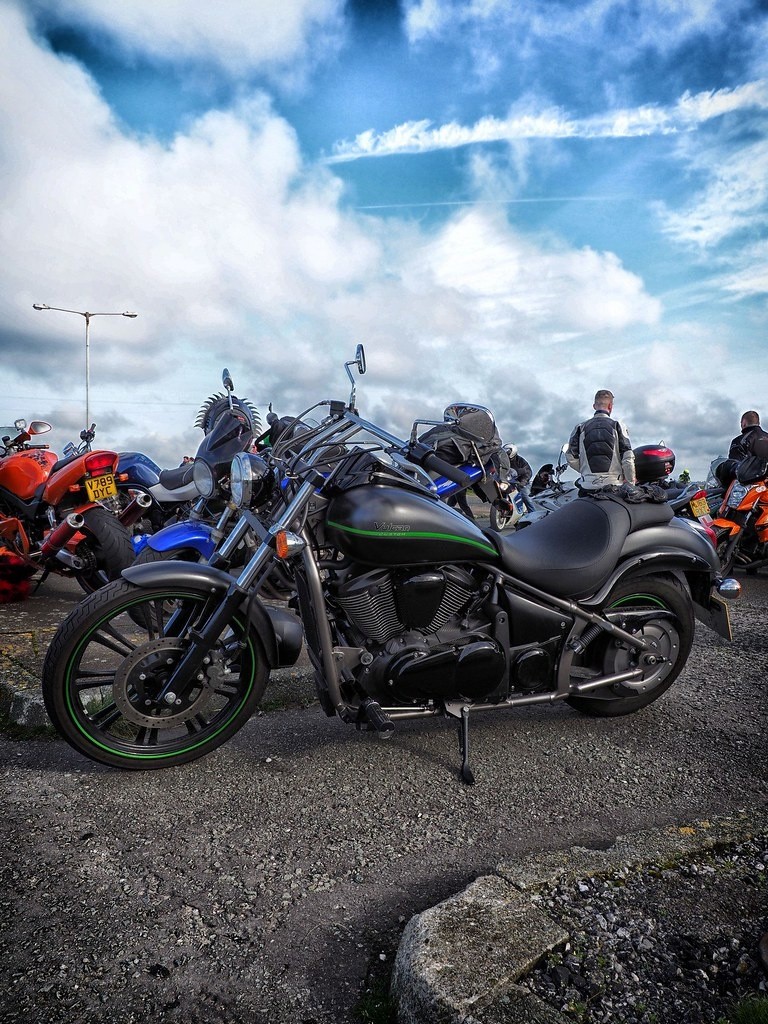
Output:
[520,480,527,487]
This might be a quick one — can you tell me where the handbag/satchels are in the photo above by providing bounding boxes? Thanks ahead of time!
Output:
[419,402,501,465]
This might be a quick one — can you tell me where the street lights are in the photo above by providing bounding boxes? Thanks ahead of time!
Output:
[32,303,138,435]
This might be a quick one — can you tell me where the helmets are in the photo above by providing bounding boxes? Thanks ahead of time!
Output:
[193,392,263,438]
[684,469,690,477]
[503,443,518,458]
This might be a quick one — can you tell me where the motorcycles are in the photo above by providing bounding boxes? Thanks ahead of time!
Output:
[0,345,767,785]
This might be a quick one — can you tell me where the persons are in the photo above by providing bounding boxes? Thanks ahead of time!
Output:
[503,443,537,512]
[179,457,194,468]
[704,411,768,490]
[564,390,636,498]
[679,469,690,483]
